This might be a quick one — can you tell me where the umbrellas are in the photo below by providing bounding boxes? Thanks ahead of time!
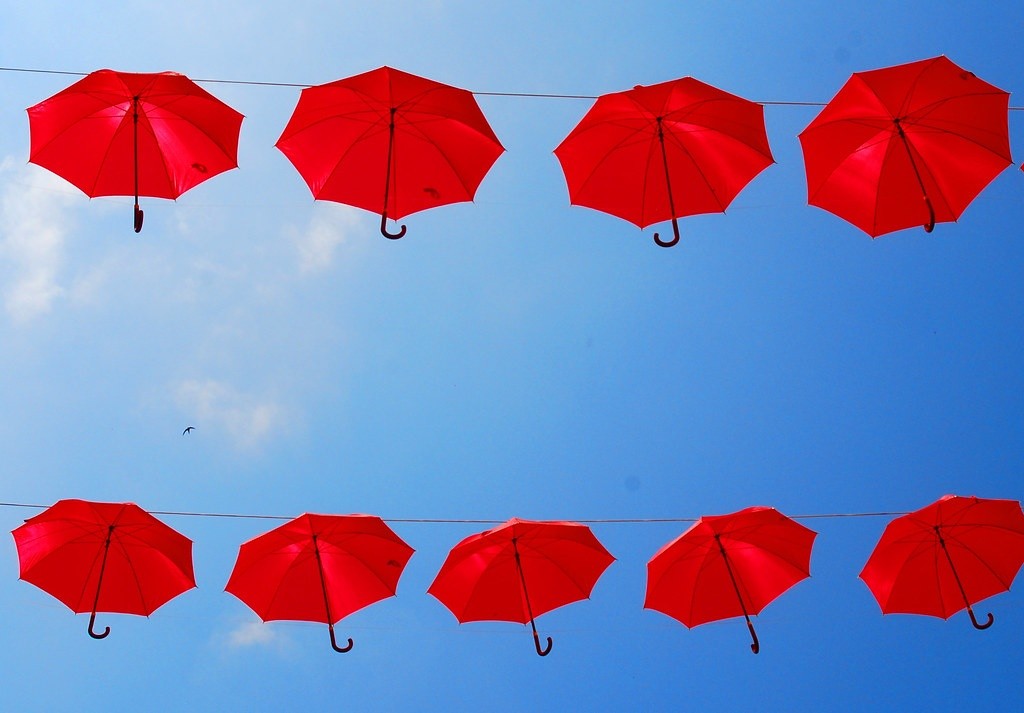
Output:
[9,498,198,639]
[552,75,778,248]
[274,66,506,240]
[643,506,819,654]
[222,512,417,653]
[426,518,618,657]
[26,69,246,232]
[797,53,1015,239]
[858,495,1024,629]
